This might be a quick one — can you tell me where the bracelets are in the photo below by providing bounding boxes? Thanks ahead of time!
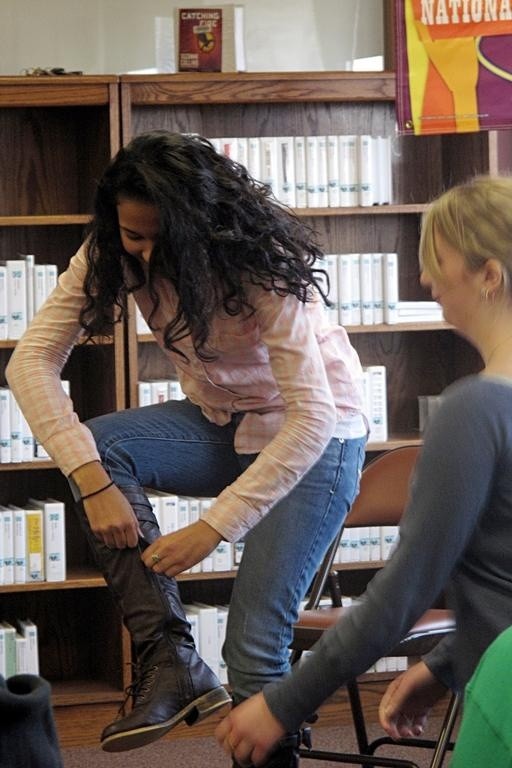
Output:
[77,480,119,501]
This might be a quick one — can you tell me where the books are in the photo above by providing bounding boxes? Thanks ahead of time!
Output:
[303,253,453,325]
[137,309,190,408]
[364,364,443,442]
[142,489,244,574]
[335,526,400,564]
[185,601,231,684]
[0,250,76,678]
[208,133,394,207]
[297,592,411,671]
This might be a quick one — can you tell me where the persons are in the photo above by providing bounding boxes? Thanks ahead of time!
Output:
[4,130,369,768]
[212,176,512,765]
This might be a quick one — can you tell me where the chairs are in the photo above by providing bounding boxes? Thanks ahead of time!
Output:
[287,444,458,766]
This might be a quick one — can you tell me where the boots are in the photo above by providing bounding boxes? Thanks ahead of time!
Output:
[231,728,311,768]
[74,488,233,751]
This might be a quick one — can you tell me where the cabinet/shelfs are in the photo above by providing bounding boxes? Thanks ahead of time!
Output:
[137,69,495,727]
[1,69,137,744]
